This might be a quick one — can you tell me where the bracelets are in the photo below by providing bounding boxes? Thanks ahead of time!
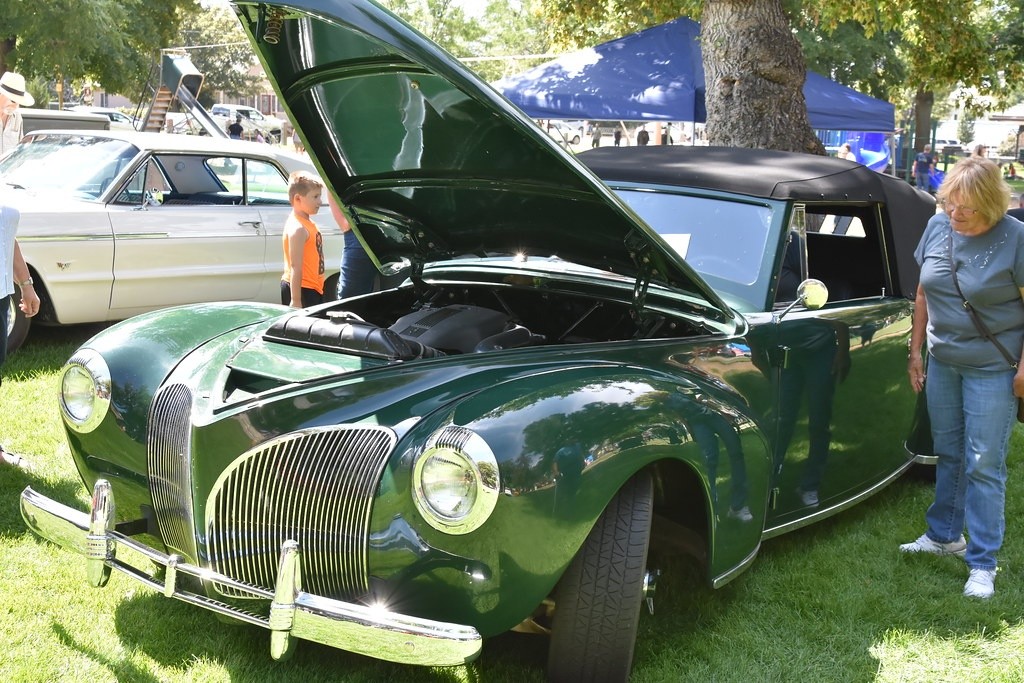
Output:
[18,277,33,286]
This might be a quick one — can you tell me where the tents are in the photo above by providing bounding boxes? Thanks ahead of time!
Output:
[487,16,898,175]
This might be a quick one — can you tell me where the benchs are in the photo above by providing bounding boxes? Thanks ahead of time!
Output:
[187,192,289,204]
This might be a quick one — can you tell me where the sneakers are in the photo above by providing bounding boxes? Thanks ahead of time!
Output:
[726,505,754,520]
[796,485,820,508]
[964,568,996,599]
[899,533,968,555]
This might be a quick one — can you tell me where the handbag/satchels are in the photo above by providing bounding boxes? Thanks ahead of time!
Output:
[1017,398,1024,423]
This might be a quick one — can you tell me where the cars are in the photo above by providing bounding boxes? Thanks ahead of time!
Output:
[20,0,938,683]
[0,129,344,356]
[935,138,957,154]
[61,106,140,129]
[535,120,582,145]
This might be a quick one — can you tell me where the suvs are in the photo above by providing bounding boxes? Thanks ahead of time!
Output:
[212,104,284,143]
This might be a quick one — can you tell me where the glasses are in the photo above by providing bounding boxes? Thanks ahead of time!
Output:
[10,100,15,104]
[941,199,981,216]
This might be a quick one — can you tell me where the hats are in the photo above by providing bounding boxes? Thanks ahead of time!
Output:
[0,71,35,106]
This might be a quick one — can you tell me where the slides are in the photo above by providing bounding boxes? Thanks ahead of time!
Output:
[163,53,231,139]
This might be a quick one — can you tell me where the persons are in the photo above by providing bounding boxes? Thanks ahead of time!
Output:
[971,144,986,158]
[898,158,1024,597]
[1006,194,1024,222]
[590,123,601,148]
[292,129,303,153]
[226,118,245,140]
[613,125,620,146]
[552,317,879,529]
[1006,163,1015,180]
[662,129,673,145]
[281,171,324,308]
[911,144,933,192]
[636,125,649,146]
[326,191,411,301]
[254,129,265,143]
[0,72,35,156]
[839,143,857,163]
[0,202,40,385]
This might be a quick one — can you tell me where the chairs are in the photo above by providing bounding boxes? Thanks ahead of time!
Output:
[166,198,216,205]
[118,192,149,204]
